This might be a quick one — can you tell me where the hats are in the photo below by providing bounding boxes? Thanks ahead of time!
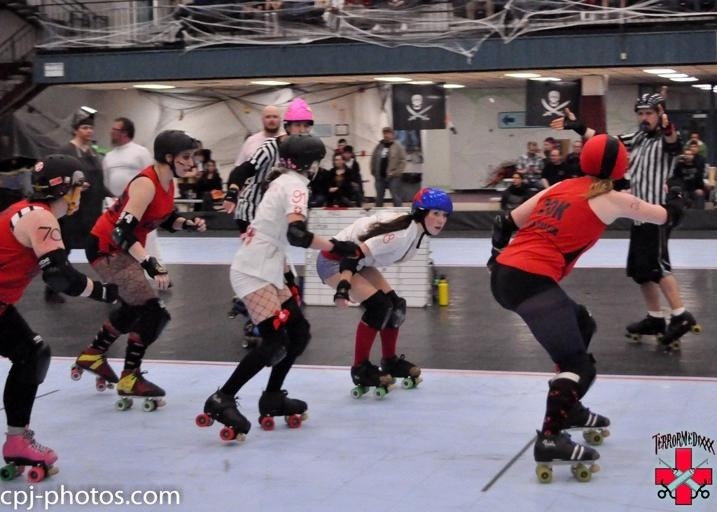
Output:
[383,127,392,132]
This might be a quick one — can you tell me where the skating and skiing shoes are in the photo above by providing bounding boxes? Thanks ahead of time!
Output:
[534,401,610,484]
[351,354,422,399]
[242,322,263,350]
[196,386,251,441]
[227,295,249,320]
[625,310,703,351]
[0,430,60,483]
[71,345,167,412]
[259,390,308,430]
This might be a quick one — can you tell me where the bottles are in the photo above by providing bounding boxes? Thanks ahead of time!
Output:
[433,273,449,306]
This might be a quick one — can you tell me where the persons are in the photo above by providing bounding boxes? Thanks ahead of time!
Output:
[220,96,315,311]
[102,116,174,289]
[1,154,120,464]
[75,127,200,396]
[368,126,405,207]
[315,186,453,388]
[203,132,361,433]
[548,92,696,343]
[309,138,365,209]
[668,129,716,209]
[232,105,285,166]
[174,140,224,215]
[500,137,583,210]
[484,134,685,462]
[43,105,114,305]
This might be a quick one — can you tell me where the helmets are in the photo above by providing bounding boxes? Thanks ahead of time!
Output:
[27,154,90,202]
[580,134,629,181]
[412,187,452,216]
[279,134,326,170]
[283,97,314,125]
[634,91,666,112]
[73,105,98,130]
[154,129,198,163]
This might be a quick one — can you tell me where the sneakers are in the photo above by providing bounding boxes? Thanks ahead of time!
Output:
[44,286,64,303]
